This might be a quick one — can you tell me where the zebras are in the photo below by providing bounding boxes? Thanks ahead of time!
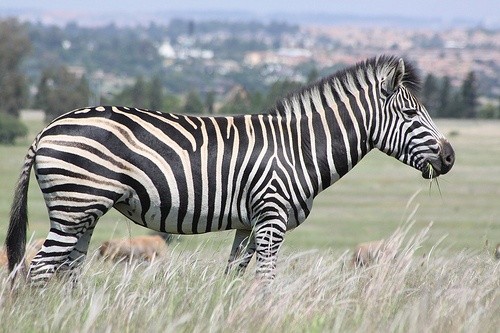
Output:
[4,53,456,305]
